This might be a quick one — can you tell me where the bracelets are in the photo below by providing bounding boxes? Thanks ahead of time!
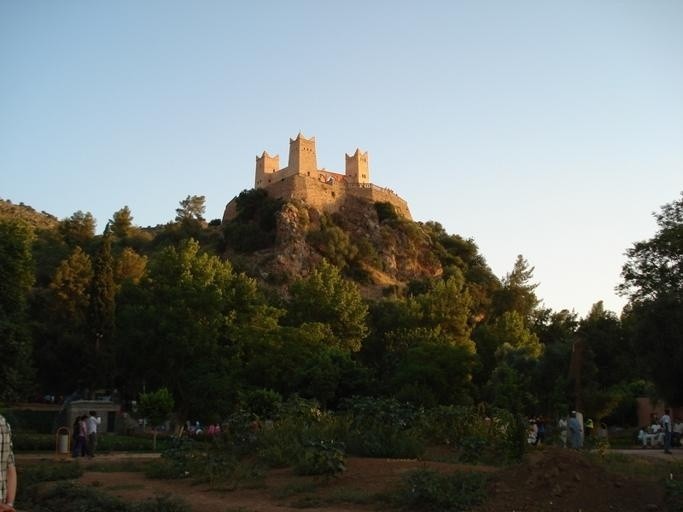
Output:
[7,501,14,508]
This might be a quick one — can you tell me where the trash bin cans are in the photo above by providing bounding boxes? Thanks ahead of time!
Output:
[57,426,70,455]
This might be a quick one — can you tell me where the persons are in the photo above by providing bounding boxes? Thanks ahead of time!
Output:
[481,406,683,457]
[1,413,19,512]
[72,409,275,459]
